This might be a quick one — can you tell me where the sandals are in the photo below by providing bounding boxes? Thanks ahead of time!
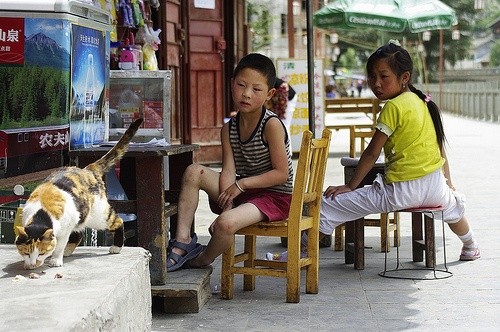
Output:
[460,247,481,260]
[180,244,215,269]
[266,249,308,270]
[165,231,203,271]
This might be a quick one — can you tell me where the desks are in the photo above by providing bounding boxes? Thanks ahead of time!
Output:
[341,155,436,271]
[66,142,201,287]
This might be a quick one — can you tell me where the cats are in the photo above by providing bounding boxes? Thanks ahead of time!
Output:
[14,117,144,270]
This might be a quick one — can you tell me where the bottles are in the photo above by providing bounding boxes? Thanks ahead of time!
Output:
[83,54,94,149]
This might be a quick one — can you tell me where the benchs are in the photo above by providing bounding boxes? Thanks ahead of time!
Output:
[323,99,381,158]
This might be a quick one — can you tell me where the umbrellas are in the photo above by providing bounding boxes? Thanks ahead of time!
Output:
[311,0,458,46]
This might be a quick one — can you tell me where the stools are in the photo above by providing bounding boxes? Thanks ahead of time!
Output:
[335,205,454,280]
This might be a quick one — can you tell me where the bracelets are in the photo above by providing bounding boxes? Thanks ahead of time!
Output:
[235,180,245,193]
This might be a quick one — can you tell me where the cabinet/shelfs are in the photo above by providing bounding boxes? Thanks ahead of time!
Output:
[108,70,172,230]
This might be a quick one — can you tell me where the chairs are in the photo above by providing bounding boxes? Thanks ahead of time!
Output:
[221,128,332,303]
[336,126,400,253]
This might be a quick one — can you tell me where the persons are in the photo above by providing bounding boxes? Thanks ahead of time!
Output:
[166,52,294,271]
[267,42,480,260]
